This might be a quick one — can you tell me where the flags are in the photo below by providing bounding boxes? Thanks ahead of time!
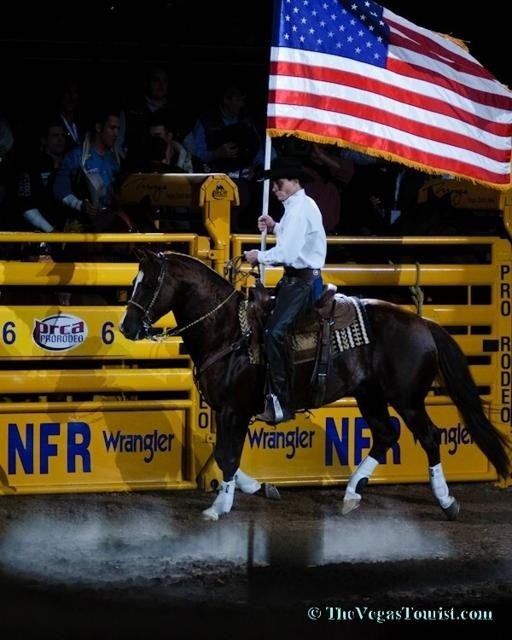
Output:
[272,0,512,193]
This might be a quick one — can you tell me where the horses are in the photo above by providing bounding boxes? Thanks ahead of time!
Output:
[119,247,512,522]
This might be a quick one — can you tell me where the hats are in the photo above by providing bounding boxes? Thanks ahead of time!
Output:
[257,158,313,183]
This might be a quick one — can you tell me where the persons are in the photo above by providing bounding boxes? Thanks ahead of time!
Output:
[0,57,512,305]
[240,156,330,425]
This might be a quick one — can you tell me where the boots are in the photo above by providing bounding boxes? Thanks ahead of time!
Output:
[256,375,292,422]
